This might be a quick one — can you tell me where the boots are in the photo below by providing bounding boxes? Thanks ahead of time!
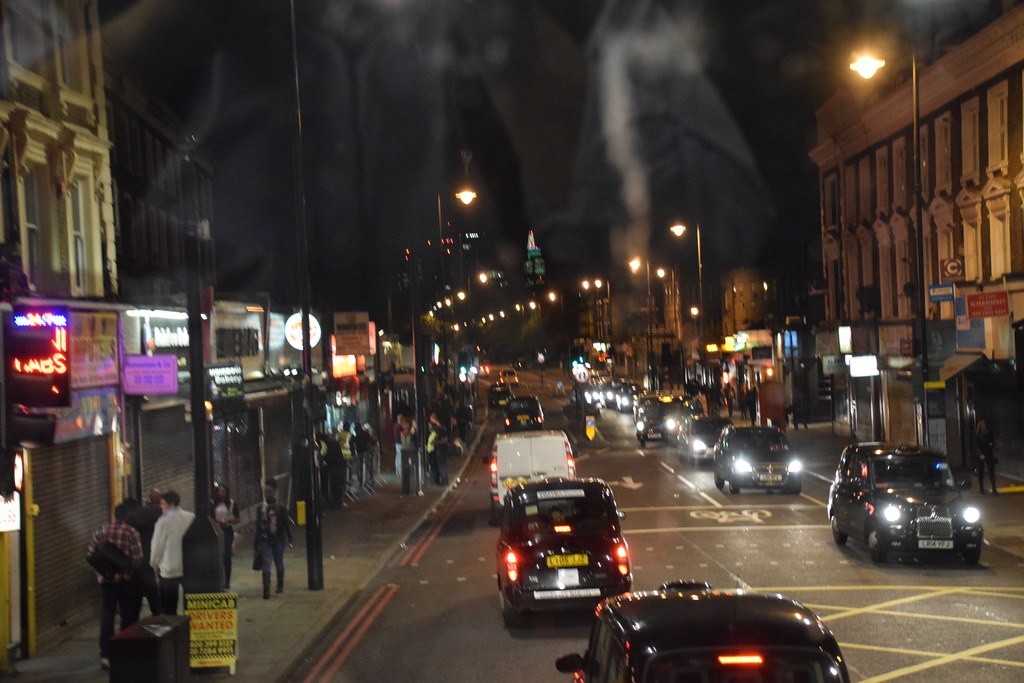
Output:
[263,571,270,598]
[276,571,284,593]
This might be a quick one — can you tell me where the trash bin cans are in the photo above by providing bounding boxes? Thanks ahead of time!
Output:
[401,448,418,495]
[109,613,190,683]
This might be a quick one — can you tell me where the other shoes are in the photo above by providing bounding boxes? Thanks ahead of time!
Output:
[99,657,109,669]
[992,490,998,496]
[980,488,984,494]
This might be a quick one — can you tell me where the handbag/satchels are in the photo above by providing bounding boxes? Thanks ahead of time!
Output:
[994,458,998,464]
[252,550,262,570]
[87,541,131,580]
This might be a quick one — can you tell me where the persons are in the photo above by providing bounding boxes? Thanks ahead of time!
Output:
[393,412,449,484]
[255,479,293,600]
[209,484,241,591]
[86,506,142,672]
[973,419,999,496]
[127,489,196,615]
[789,385,808,431]
[683,378,757,424]
[315,420,380,490]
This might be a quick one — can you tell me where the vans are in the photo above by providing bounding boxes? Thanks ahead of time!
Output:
[484,430,577,526]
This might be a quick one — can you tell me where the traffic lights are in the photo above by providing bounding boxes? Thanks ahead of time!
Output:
[1,313,56,495]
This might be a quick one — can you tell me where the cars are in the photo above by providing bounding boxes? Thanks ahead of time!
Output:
[584,369,803,495]
[488,369,544,432]
[555,579,851,682]
[828,441,983,568]
[495,479,634,629]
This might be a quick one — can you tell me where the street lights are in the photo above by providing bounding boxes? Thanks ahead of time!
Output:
[670,216,705,335]
[850,39,928,381]
[435,157,478,388]
[628,254,657,389]
[581,278,614,338]
[515,292,564,324]
